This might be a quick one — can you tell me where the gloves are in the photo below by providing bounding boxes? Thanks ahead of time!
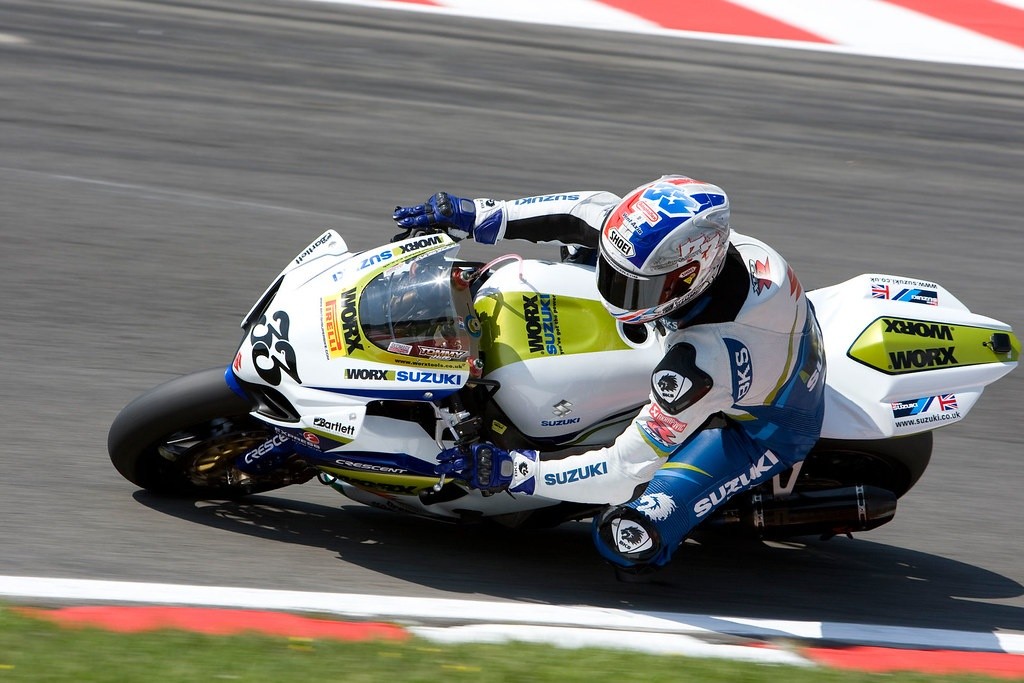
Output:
[392,191,508,245]
[433,440,541,497]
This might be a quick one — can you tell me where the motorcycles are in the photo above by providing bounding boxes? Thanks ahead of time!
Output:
[108,204,1021,546]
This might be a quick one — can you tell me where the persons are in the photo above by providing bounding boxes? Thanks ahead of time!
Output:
[393,174,826,581]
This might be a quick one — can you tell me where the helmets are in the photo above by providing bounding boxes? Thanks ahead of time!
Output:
[594,173,731,325]
